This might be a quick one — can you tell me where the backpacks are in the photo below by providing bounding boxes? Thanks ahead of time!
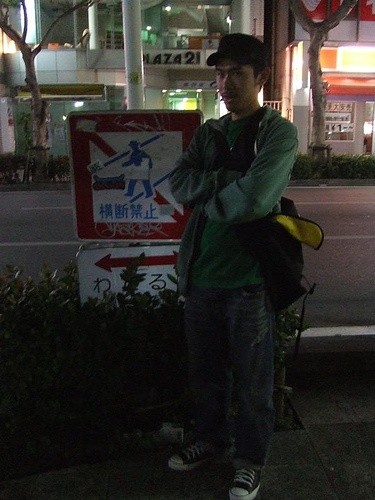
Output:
[261,196,324,314]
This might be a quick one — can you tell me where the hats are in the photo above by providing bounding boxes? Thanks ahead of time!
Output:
[205,32,269,66]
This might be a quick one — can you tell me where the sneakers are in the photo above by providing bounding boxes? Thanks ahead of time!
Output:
[166,436,217,469]
[228,464,261,499]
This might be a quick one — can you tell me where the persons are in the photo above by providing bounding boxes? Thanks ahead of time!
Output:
[78,29,89,52]
[168,33,298,500]
